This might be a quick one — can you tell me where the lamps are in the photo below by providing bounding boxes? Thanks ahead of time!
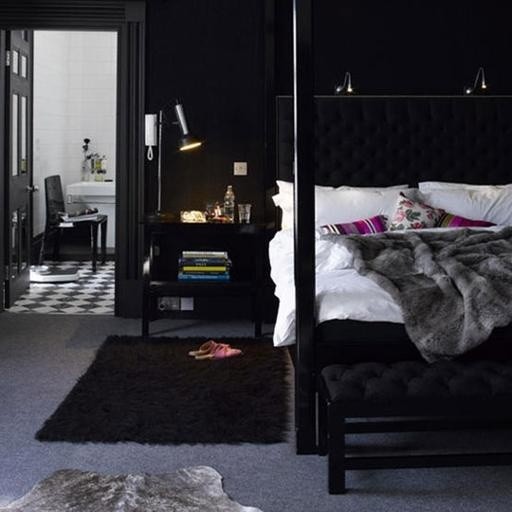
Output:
[462,66,488,96]
[333,68,356,97]
[137,94,209,224]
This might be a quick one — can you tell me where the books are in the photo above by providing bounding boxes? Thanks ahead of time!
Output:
[177,250,232,282]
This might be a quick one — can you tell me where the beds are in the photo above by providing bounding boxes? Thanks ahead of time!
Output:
[255,1,510,459]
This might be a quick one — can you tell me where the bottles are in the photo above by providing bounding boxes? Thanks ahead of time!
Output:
[224,185,234,220]
[96,154,107,172]
[82,160,90,182]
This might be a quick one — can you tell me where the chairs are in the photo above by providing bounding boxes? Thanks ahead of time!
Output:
[41,174,113,274]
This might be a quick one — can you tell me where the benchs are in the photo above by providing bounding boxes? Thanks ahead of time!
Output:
[310,347,512,495]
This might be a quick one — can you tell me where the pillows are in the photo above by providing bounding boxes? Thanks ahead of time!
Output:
[268,175,511,237]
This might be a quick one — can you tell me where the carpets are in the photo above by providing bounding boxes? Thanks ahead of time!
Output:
[33,334,292,443]
[1,467,267,511]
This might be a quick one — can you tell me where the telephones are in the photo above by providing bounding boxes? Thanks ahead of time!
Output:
[145,114,158,146]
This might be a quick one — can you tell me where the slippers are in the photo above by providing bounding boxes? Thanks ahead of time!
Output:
[189,340,231,357]
[196,346,242,361]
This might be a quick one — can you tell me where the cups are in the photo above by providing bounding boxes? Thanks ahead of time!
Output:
[96,174,104,182]
[237,204,251,224]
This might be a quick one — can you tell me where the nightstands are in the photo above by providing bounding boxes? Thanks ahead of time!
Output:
[129,214,284,339]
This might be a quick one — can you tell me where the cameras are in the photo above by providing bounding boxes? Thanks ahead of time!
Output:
[157,296,180,311]
